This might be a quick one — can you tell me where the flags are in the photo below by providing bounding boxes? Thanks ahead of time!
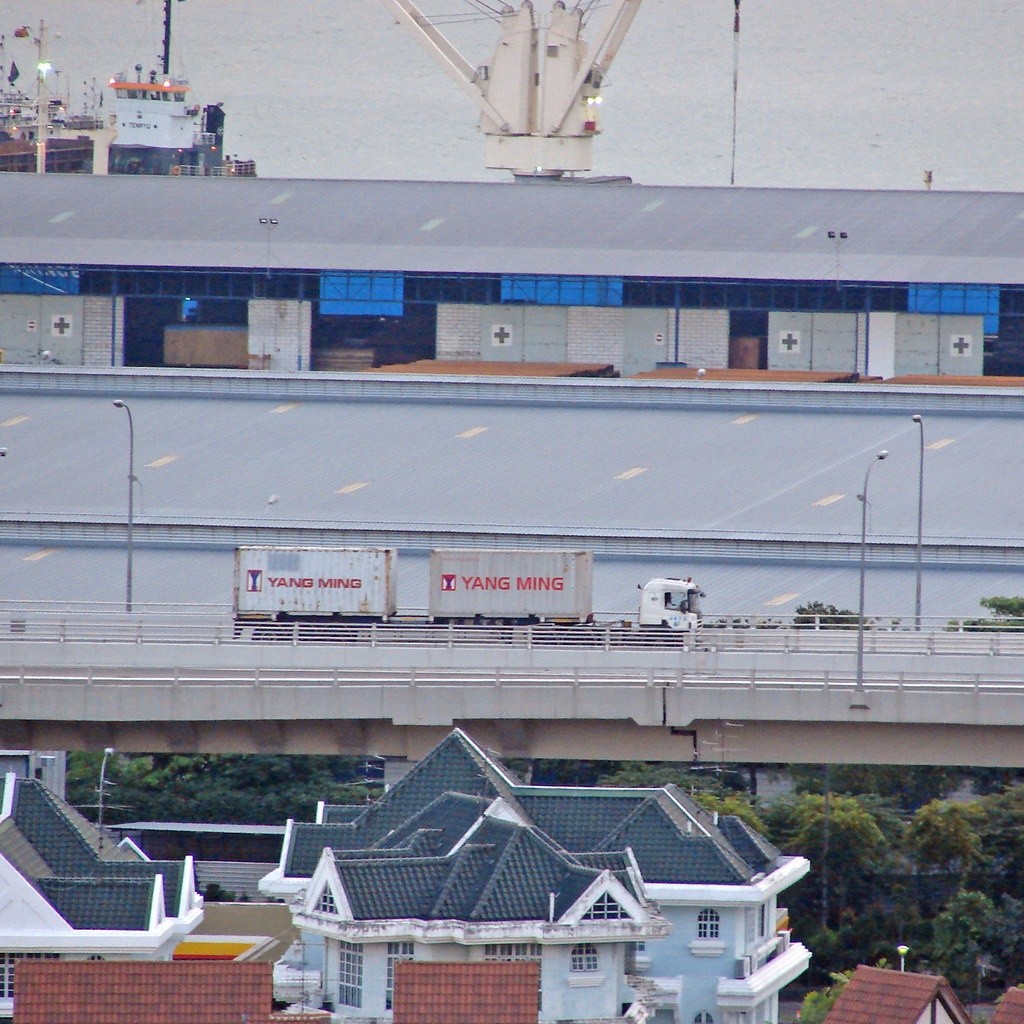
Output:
[6,61,19,81]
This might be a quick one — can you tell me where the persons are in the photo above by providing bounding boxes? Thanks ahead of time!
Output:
[665,593,680,610]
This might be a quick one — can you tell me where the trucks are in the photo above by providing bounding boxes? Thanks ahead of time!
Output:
[231,546,708,646]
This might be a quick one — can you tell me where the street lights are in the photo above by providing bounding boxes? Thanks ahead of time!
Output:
[912,414,924,629]
[113,399,134,610]
[15,20,49,172]
[855,450,890,692]
